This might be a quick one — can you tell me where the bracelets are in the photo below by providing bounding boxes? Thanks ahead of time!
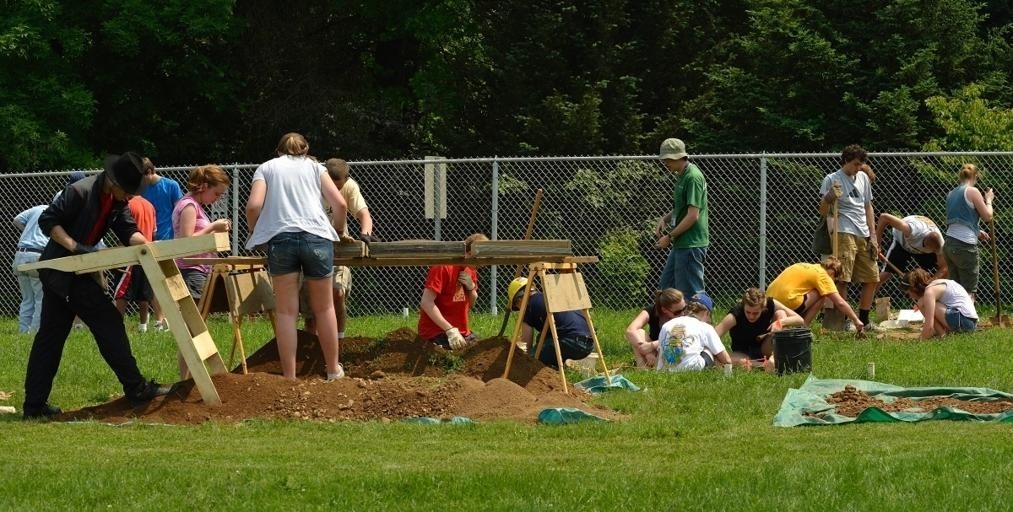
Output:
[667,231,674,243]
[986,202,992,206]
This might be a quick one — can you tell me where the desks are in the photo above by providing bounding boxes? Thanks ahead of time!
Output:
[182,253,610,396]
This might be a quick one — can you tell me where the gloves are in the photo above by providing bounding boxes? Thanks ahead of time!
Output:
[866,233,878,261]
[444,328,466,350]
[457,271,475,291]
[71,242,98,255]
[824,183,842,203]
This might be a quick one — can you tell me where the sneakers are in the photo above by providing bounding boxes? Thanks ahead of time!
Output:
[125,378,177,400]
[22,400,61,419]
[863,323,878,331]
[325,362,344,380]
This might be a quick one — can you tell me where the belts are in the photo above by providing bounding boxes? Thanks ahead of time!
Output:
[16,248,43,254]
[566,334,593,344]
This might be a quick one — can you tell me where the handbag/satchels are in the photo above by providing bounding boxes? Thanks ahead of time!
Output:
[814,215,832,255]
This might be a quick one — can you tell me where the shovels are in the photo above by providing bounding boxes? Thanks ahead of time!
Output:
[985,187,1011,328]
[822,179,845,332]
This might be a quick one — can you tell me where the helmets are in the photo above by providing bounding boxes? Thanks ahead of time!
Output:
[507,277,535,311]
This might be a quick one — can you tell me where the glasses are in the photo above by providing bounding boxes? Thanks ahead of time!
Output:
[664,306,686,316]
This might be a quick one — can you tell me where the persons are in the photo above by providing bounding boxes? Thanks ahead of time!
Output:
[655,290,733,374]
[169,162,233,380]
[941,161,995,304]
[303,157,373,339]
[12,198,55,333]
[52,171,109,294]
[623,286,688,372]
[714,286,805,374]
[246,131,348,383]
[868,211,951,310]
[765,255,865,341]
[20,153,181,423]
[654,136,711,304]
[811,163,877,323]
[819,142,888,336]
[129,156,185,330]
[418,233,491,350]
[900,268,979,342]
[506,275,595,373]
[112,194,160,334]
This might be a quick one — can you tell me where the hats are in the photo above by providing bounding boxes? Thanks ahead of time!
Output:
[103,151,145,196]
[689,294,712,311]
[659,138,687,161]
[65,172,85,185]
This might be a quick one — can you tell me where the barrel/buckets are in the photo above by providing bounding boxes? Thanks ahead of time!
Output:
[772,329,813,373]
